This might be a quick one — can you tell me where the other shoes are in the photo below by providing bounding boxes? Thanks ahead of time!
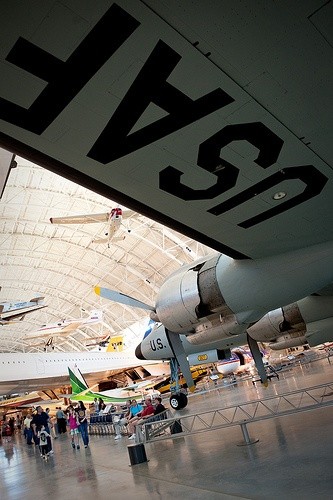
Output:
[114,434,122,440]
[40,443,89,458]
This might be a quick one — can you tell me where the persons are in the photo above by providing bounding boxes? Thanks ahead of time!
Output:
[0,398,165,461]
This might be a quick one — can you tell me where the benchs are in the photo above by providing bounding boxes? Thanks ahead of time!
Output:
[252,375,279,384]
[89,410,166,434]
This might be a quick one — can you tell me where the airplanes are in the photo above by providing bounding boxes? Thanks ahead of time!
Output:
[135,284,332,410]
[68,363,161,412]
[0,0,333,347]
[215,345,289,375]
[23,304,104,351]
[0,286,48,325]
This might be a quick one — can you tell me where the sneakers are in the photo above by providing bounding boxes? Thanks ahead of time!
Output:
[128,433,136,440]
